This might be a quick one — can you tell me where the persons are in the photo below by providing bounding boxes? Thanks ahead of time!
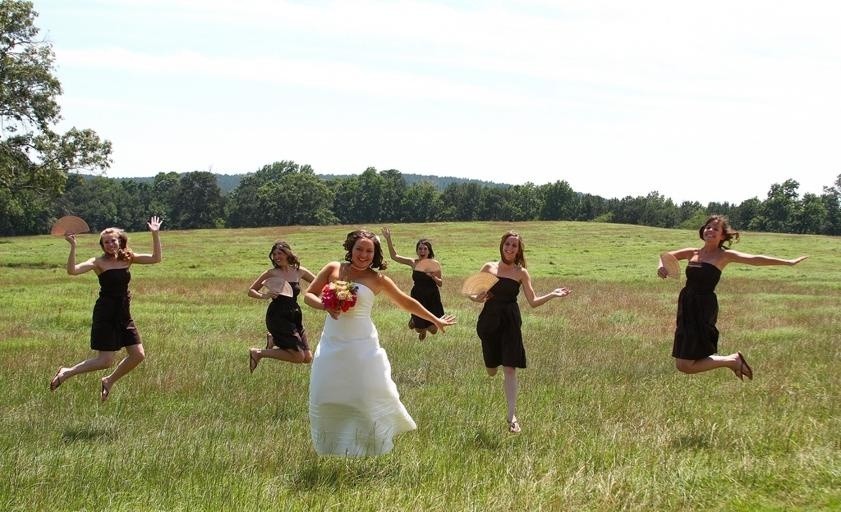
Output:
[382,227,445,341]
[248,240,316,374]
[305,229,457,459]
[657,215,809,382]
[48,214,164,402]
[468,231,574,433]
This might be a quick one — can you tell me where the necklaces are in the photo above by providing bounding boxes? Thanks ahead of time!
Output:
[350,263,368,271]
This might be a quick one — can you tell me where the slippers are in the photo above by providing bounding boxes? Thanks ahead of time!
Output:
[735,369,743,382]
[101,379,109,401]
[266,332,273,350]
[507,420,521,433]
[737,351,753,380]
[249,349,257,374]
[49,366,64,392]
